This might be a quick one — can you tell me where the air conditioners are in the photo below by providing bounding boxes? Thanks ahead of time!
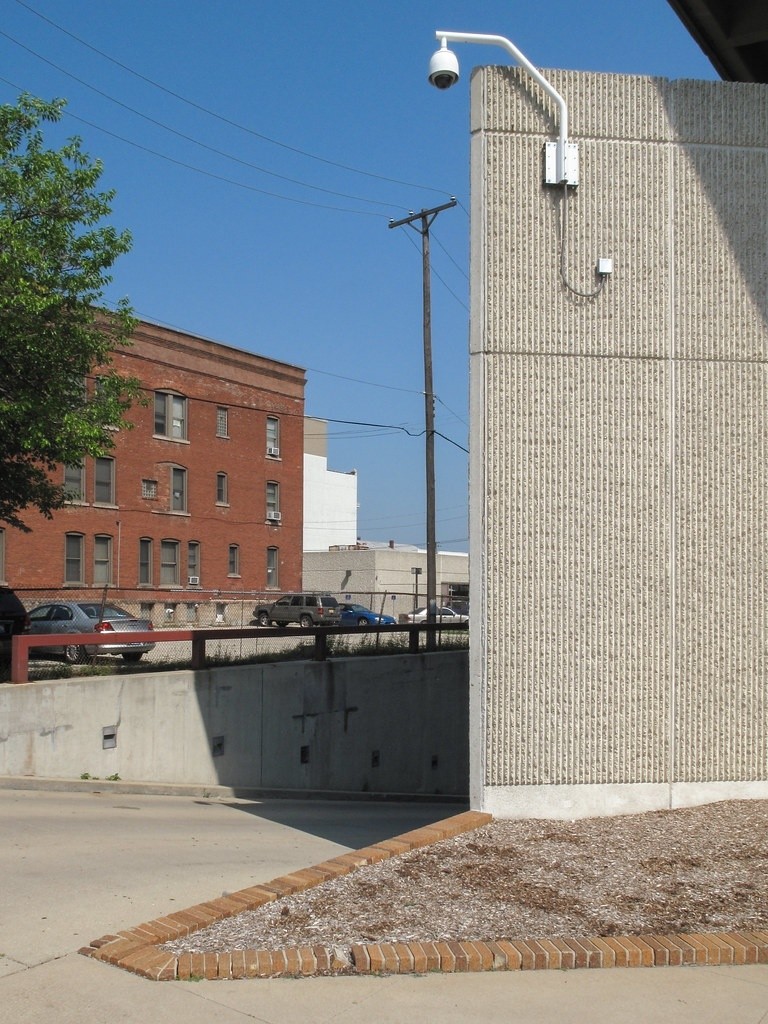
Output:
[188,576,200,584]
[267,447,279,456]
[266,511,281,520]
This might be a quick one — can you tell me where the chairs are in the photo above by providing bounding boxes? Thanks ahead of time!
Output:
[51,608,69,620]
[85,608,95,617]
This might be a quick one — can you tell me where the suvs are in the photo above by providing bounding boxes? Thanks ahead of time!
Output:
[253,594,342,627]
[0,585,31,683]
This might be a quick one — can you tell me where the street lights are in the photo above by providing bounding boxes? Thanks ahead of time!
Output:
[410,567,422,609]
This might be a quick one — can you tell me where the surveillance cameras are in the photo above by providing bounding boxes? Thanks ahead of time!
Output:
[427,49,459,90]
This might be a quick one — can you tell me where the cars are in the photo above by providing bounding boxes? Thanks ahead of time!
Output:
[26,601,156,664]
[338,602,398,627]
[398,607,470,624]
